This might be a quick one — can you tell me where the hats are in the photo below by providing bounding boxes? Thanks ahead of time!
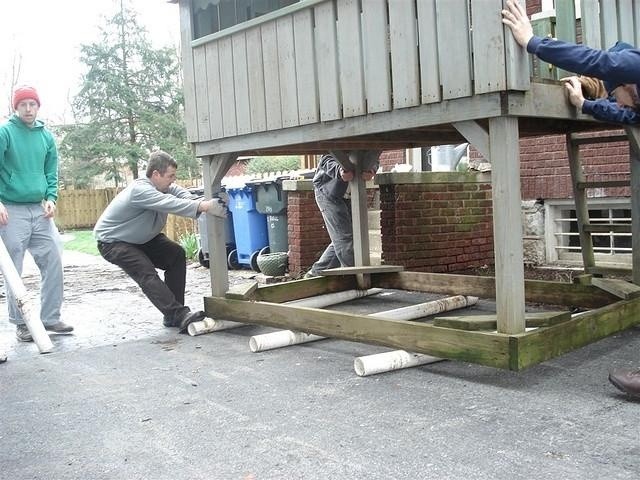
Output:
[13,84,41,111]
[601,42,634,94]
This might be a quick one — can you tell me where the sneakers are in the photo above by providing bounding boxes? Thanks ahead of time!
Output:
[15,324,34,342]
[163,310,204,333]
[607,365,639,401]
[303,272,312,280]
[43,322,73,333]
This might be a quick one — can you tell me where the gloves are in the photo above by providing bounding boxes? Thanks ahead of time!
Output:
[214,189,229,205]
[207,199,228,218]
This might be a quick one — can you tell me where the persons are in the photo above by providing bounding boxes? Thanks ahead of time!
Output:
[93,152,229,336]
[0,84,74,343]
[302,150,382,279]
[501,0,640,402]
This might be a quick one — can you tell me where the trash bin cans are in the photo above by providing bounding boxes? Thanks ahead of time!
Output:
[192,175,302,273]
